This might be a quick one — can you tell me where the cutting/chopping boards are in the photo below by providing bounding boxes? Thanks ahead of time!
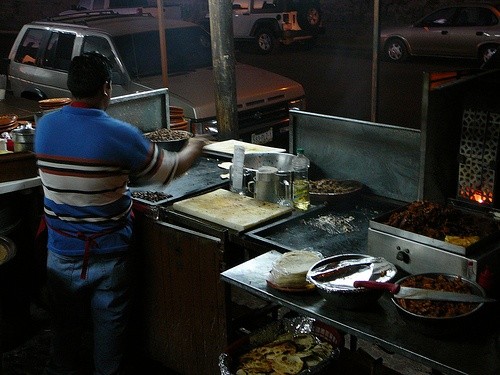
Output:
[173,188,292,231]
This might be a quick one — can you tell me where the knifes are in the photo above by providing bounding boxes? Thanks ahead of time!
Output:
[354,280,498,303]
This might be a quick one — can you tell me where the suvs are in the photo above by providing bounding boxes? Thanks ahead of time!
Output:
[58,0,326,56]
[4,9,307,146]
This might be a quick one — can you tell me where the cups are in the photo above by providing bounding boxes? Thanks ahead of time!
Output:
[13,127,35,153]
[248,167,280,203]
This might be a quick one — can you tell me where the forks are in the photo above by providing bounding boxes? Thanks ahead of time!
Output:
[307,254,397,304]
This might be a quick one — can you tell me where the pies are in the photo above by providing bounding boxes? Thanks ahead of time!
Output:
[444,235,479,248]
[236,333,333,375]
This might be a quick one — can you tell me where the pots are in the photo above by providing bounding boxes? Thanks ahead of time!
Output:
[230,152,311,199]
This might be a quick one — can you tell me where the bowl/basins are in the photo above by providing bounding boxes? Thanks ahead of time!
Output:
[0,234,17,266]
[390,271,487,327]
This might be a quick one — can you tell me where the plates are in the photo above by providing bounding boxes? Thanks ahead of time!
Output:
[308,178,362,196]
[202,138,286,160]
[265,279,316,292]
[143,131,192,143]
[169,106,189,132]
[0,96,72,138]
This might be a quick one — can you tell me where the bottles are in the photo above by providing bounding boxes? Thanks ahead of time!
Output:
[291,147,311,209]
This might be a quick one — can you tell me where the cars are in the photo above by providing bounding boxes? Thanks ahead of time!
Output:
[379,3,500,68]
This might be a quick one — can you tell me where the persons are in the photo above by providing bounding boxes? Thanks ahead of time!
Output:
[34,52,207,375]
